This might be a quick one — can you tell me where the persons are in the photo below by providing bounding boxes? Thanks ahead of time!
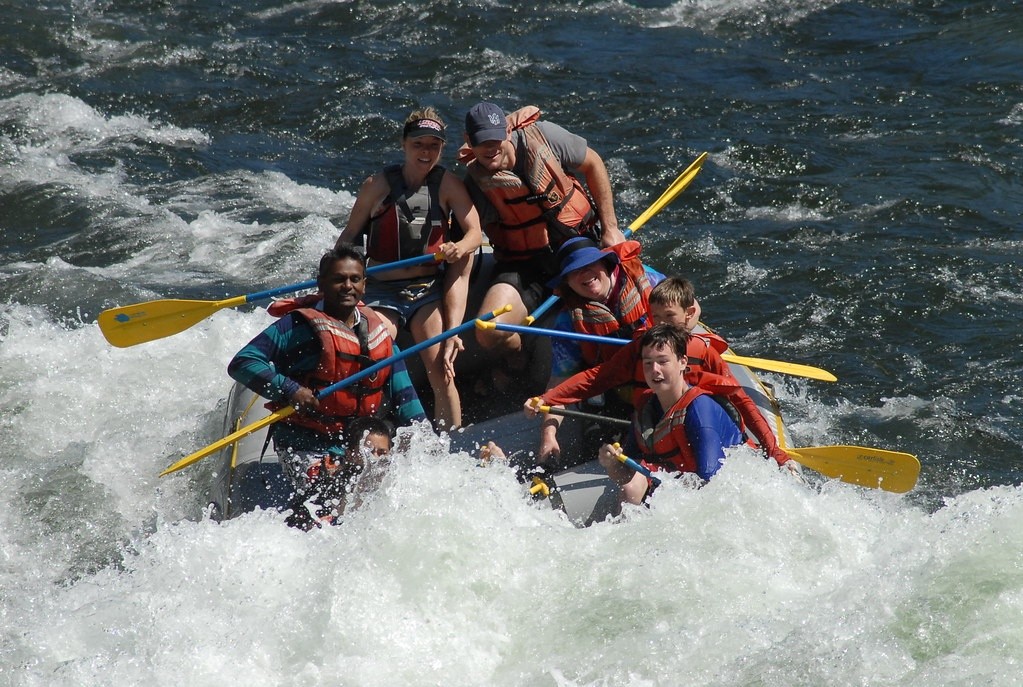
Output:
[227,240,428,533]
[523,237,801,509]
[441,101,627,412]
[316,104,482,434]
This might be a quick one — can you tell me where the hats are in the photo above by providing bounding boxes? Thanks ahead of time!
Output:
[403,119,446,142]
[466,101,508,147]
[545,237,621,289]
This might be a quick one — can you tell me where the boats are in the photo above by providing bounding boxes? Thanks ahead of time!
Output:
[218,244,803,531]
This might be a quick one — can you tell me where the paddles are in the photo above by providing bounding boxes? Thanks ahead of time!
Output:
[606,439,665,488]
[527,393,922,495]
[158,304,840,478]
[524,151,708,326]
[98,250,447,348]
